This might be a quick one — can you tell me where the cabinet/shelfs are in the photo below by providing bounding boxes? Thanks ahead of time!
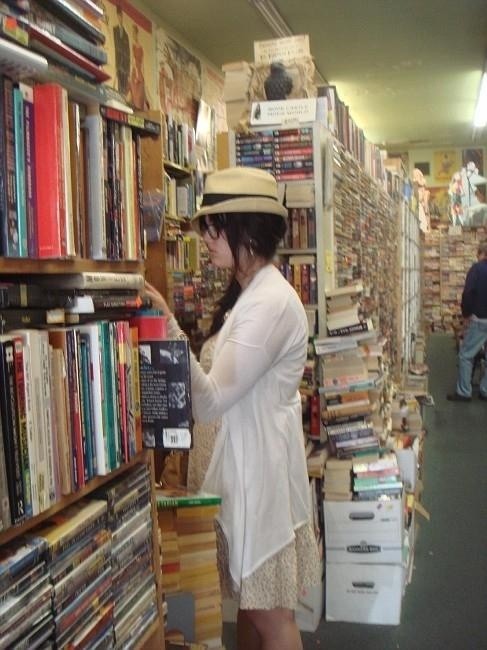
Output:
[0,46,428,650]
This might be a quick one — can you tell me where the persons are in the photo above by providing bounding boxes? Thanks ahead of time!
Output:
[445,240,486,403]
[146,167,318,649]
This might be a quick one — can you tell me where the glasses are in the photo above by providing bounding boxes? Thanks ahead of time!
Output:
[201,224,231,240]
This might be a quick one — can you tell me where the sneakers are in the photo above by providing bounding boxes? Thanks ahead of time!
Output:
[447,393,471,401]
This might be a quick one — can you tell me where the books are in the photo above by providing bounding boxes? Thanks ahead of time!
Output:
[235,127,328,481]
[317,85,432,528]
[2,0,221,650]
[423,230,487,329]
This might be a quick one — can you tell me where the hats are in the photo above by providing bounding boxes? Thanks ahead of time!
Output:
[189,166,288,236]
